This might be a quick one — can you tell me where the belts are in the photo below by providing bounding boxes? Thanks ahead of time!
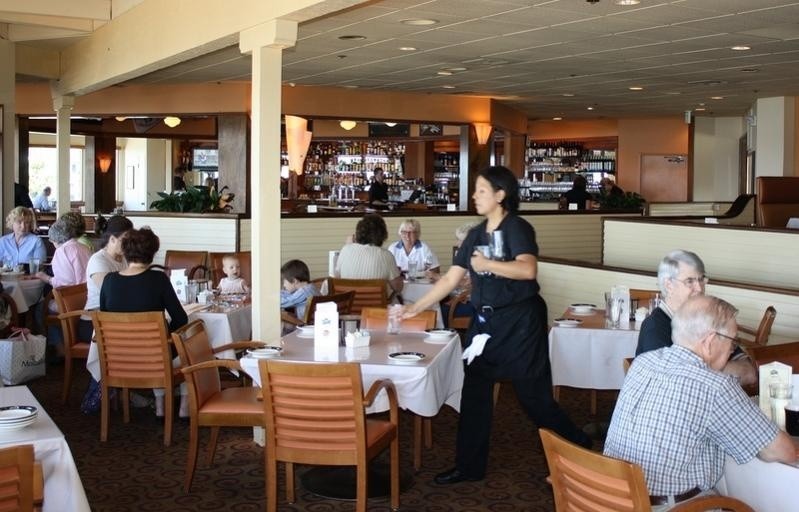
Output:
[472,304,510,313]
[649,487,702,506]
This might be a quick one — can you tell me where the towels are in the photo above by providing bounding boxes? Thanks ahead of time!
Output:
[460,333,491,366]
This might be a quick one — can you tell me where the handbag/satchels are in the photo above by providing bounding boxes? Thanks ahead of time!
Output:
[0,329,47,385]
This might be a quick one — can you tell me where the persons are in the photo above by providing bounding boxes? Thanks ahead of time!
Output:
[601,178,625,199]
[388,220,440,282]
[389,167,591,484]
[561,176,592,210]
[635,251,760,385]
[30,213,94,326]
[81,217,153,408]
[99,227,189,422]
[174,167,187,192]
[216,255,249,305]
[33,188,55,213]
[603,296,798,512]
[335,215,403,297]
[281,261,322,324]
[426,222,484,328]
[369,168,396,211]
[1,203,47,337]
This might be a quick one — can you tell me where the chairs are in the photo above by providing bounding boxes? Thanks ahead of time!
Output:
[87,311,175,449]
[60,278,96,410]
[537,428,755,512]
[277,291,359,334]
[319,273,396,319]
[256,361,403,511]
[159,248,252,296]
[172,322,266,497]
[623,288,799,396]
[360,304,442,345]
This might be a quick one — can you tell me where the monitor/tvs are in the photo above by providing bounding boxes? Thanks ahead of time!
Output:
[409,189,422,201]
[191,146,218,171]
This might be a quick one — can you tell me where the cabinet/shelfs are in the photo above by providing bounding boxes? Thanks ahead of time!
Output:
[281,136,407,192]
[526,134,619,192]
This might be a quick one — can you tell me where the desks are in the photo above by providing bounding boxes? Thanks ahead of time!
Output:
[0,263,52,319]
[240,321,466,495]
[715,396,799,512]
[147,294,252,414]
[0,377,93,512]
[548,306,645,415]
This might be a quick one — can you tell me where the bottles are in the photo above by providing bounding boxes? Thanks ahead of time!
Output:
[304,142,401,186]
[331,185,356,200]
[439,153,458,173]
[281,155,288,165]
[418,187,459,206]
[527,139,615,193]
[654,292,660,309]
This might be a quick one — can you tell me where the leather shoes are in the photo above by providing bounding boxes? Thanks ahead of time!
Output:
[434,468,485,484]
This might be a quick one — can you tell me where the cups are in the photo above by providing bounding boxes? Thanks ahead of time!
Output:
[29,258,39,275]
[185,279,198,304]
[387,304,403,335]
[607,298,623,328]
[407,259,419,278]
[476,230,504,275]
[634,307,647,321]
[634,321,645,331]
[784,404,799,437]
[329,195,337,207]
[768,383,794,426]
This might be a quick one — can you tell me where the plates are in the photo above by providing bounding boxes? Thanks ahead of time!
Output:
[249,353,281,359]
[0,405,39,432]
[246,344,285,355]
[570,304,597,309]
[297,323,319,331]
[1,272,25,278]
[424,337,452,345]
[553,318,583,327]
[295,331,315,339]
[571,309,597,317]
[387,351,427,364]
[423,328,457,337]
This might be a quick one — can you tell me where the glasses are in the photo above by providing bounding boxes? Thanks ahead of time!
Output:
[702,332,742,349]
[670,276,710,287]
